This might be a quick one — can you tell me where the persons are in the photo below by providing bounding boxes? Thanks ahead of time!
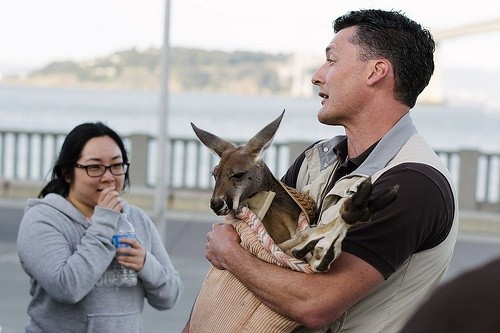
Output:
[204,9,460,333]
[16,122,184,333]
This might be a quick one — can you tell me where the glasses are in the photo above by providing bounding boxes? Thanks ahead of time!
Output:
[74,162,130,178]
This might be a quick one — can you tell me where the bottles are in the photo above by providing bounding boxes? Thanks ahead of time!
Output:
[113,213,138,287]
